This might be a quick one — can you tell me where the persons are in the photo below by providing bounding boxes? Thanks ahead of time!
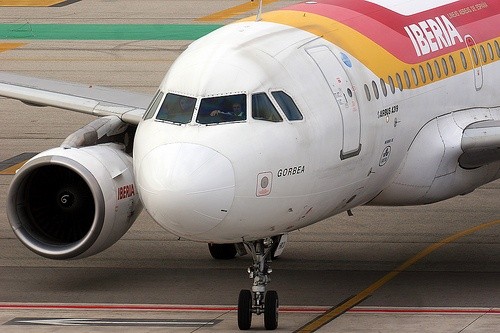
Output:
[210,101,246,118]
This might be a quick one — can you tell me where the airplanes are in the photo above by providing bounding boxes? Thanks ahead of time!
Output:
[0,1,500,330]
